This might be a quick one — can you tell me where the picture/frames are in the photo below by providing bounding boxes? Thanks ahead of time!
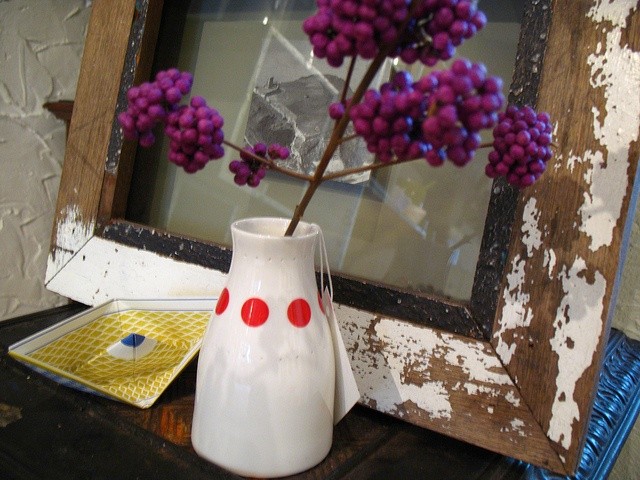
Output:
[42,0,640,477]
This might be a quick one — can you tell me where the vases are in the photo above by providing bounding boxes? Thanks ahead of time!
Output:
[189,216,336,478]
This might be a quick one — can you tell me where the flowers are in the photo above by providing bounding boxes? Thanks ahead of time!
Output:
[116,0,560,237]
